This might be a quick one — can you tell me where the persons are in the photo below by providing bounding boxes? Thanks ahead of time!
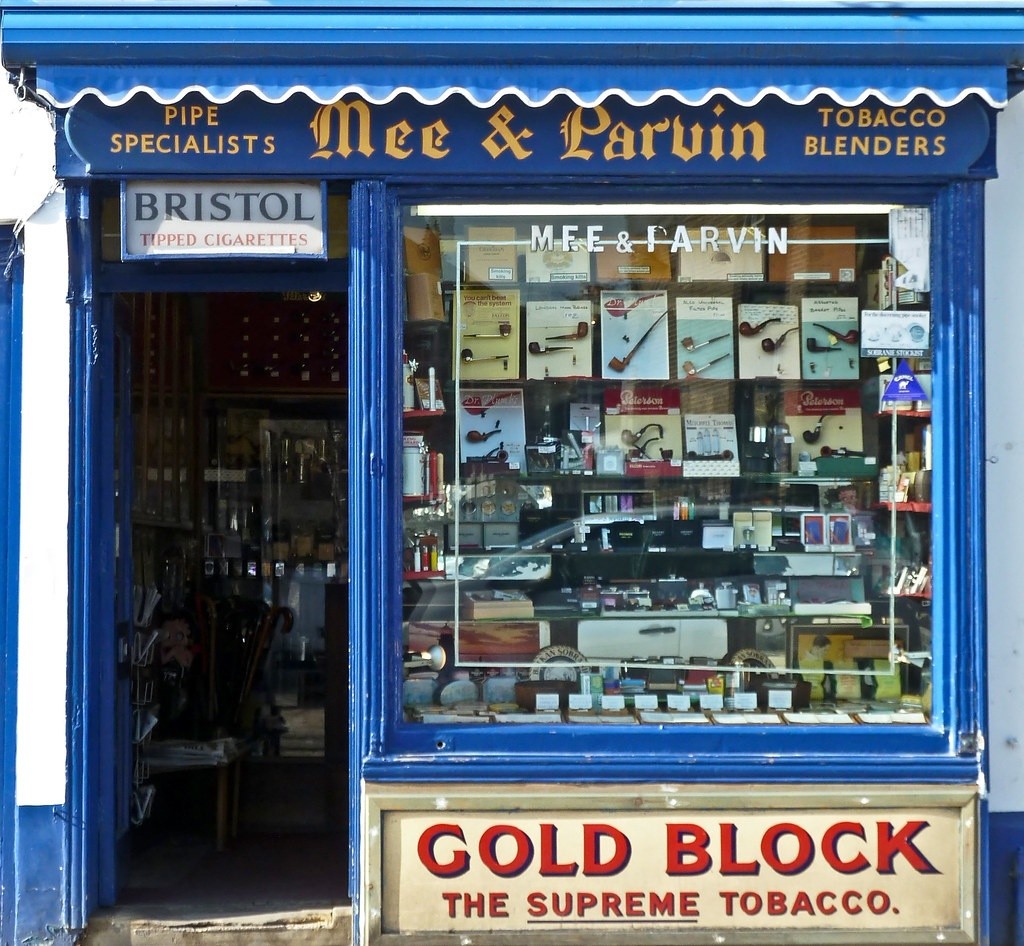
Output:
[802,634,831,662]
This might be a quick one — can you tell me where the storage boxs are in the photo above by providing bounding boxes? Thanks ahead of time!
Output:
[810,457,880,477]
[513,680,578,711]
[403,226,446,320]
[877,467,903,502]
[459,653,538,663]
[444,553,552,580]
[768,225,857,281]
[408,620,551,653]
[676,227,764,280]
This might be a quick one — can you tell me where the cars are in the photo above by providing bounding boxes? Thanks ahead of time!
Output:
[394,499,926,698]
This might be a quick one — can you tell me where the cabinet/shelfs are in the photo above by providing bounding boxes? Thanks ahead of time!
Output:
[887,258,933,599]
[405,281,890,626]
[404,408,447,583]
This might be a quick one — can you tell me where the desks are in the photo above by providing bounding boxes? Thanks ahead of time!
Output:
[140,747,249,853]
